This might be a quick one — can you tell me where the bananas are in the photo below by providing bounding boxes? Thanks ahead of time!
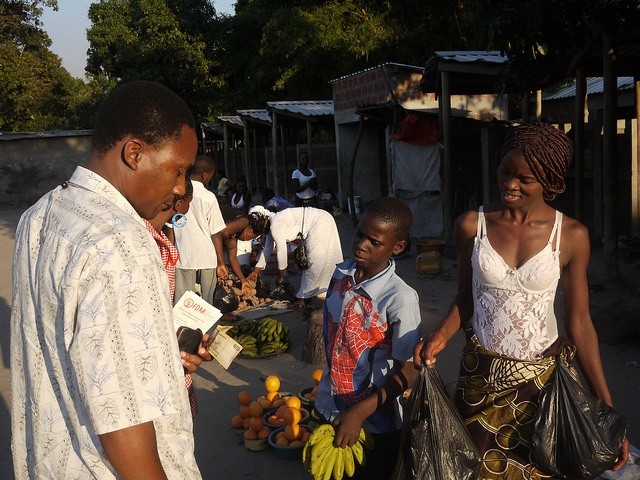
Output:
[257,318,289,342]
[302,422,374,480]
[219,321,240,338]
[239,318,258,336]
[262,341,289,354]
[232,334,258,358]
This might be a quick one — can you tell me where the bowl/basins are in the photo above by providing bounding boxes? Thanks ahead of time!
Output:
[268,424,314,459]
[262,407,310,430]
[298,387,320,404]
[240,427,272,451]
[311,405,320,420]
[262,391,295,412]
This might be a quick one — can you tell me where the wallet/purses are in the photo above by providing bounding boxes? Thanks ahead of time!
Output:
[177,327,203,374]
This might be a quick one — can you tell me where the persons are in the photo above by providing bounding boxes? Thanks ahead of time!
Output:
[313,197,423,479]
[291,152,318,207]
[214,169,229,203]
[248,205,344,310]
[221,206,263,295]
[174,156,228,334]
[414,124,629,478]
[143,174,193,307]
[10,81,204,479]
[261,188,294,212]
[230,178,252,209]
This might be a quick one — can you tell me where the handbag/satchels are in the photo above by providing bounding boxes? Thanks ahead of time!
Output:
[214,283,239,313]
[294,232,307,269]
